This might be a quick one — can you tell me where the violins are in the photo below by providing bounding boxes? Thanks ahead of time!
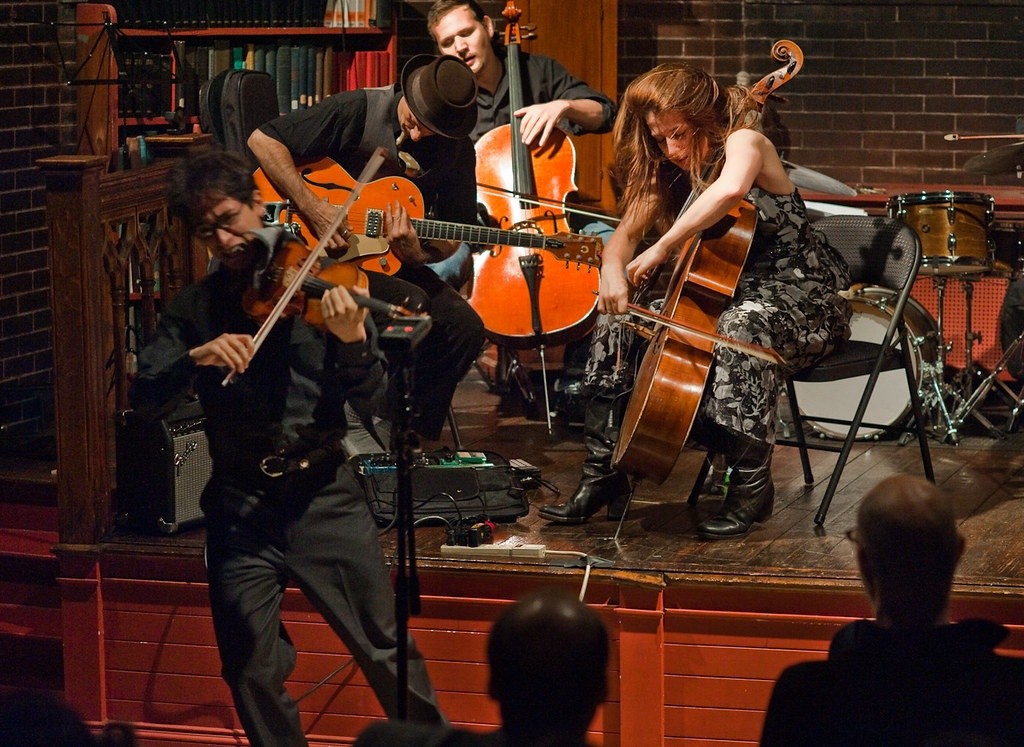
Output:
[241,238,430,334]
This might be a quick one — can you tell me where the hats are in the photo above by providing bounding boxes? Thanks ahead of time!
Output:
[400,53,478,138]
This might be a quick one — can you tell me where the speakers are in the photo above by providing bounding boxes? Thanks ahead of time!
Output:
[114,396,213,535]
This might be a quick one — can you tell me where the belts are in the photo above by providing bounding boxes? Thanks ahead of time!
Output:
[211,439,346,477]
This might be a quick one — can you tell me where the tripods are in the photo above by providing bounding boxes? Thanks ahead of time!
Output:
[898,268,1024,446]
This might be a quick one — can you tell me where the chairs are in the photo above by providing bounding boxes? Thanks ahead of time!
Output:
[691,214,934,526]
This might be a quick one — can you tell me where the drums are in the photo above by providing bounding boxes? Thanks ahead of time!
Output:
[789,286,946,442]
[887,189,997,279]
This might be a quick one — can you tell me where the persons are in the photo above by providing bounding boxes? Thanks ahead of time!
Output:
[757,476,1024,747]
[130,152,457,747]
[352,594,612,747]
[248,55,485,465]
[538,60,851,540]
[426,0,618,416]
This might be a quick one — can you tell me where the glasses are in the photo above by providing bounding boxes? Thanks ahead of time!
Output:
[192,202,243,241]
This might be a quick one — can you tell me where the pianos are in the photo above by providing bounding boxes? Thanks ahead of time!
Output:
[947,218,1024,440]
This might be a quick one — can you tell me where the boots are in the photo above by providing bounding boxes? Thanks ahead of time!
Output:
[536,392,631,522]
[698,434,773,540]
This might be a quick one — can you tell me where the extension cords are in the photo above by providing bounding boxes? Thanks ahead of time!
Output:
[441,543,547,559]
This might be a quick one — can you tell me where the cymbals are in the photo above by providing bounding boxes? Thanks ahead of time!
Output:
[779,160,859,197]
[961,141,1024,177]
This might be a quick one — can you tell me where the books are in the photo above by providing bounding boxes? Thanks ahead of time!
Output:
[117,36,389,118]
[119,247,192,296]
[90,1,394,29]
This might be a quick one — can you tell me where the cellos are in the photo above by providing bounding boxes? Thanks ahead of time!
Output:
[464,0,603,438]
[604,38,806,543]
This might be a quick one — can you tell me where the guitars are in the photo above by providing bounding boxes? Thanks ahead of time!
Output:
[250,153,604,282]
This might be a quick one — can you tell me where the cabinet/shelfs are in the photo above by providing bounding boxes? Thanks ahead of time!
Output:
[71,0,397,178]
